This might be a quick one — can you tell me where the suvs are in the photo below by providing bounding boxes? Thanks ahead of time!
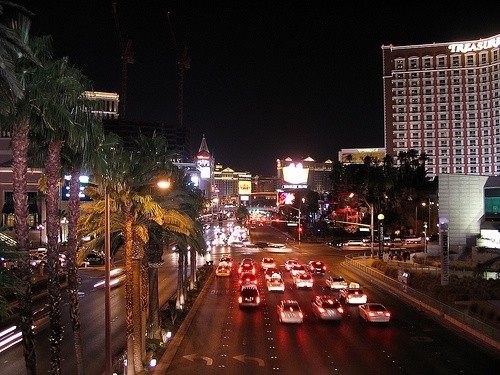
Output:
[237,284,261,310]
[293,272,314,290]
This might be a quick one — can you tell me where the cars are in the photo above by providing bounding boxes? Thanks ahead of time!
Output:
[339,282,367,305]
[265,268,281,279]
[312,295,343,322]
[261,257,275,269]
[277,300,303,325]
[238,257,256,275]
[327,237,423,248]
[284,260,297,272]
[358,303,390,324]
[30,246,105,268]
[289,264,305,279]
[215,254,233,278]
[239,272,257,286]
[325,276,347,291]
[265,277,285,293]
[306,261,326,276]
[201,212,292,253]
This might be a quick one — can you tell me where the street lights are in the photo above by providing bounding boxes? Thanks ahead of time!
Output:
[377,213,385,260]
[106,180,172,375]
[349,192,374,258]
[298,197,306,246]
[415,202,426,238]
